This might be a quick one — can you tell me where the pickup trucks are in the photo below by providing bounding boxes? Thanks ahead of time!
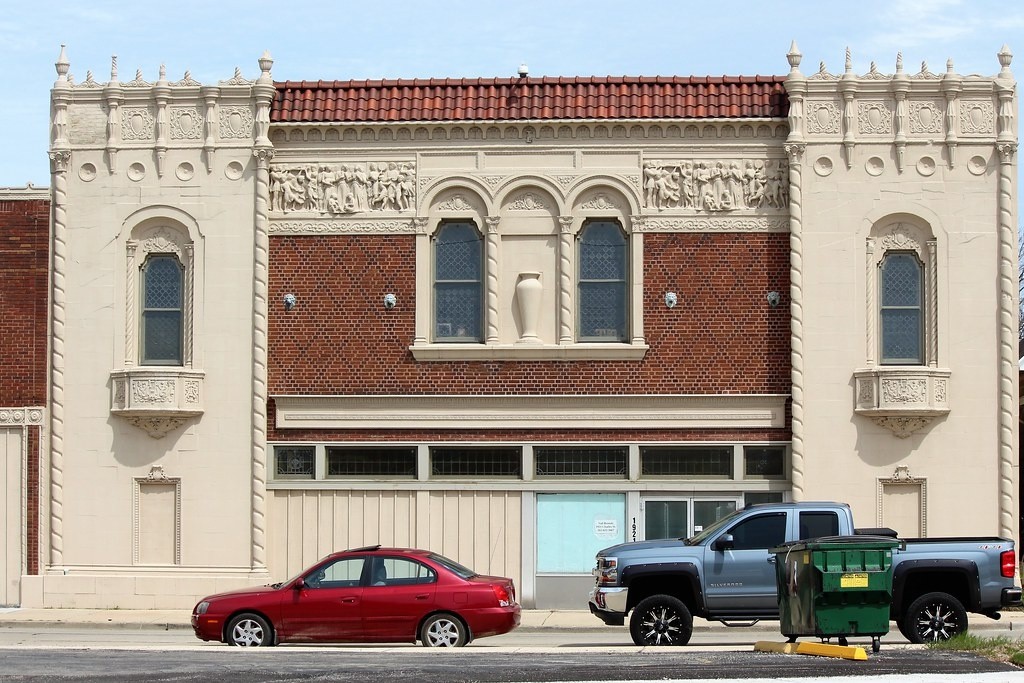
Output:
[587,500,1024,646]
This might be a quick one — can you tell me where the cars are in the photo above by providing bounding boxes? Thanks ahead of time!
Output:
[190,546,522,647]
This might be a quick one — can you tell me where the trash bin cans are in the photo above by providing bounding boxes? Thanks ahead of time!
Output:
[768,534,907,653]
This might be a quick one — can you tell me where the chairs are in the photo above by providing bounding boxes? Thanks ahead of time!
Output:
[373,566,387,586]
